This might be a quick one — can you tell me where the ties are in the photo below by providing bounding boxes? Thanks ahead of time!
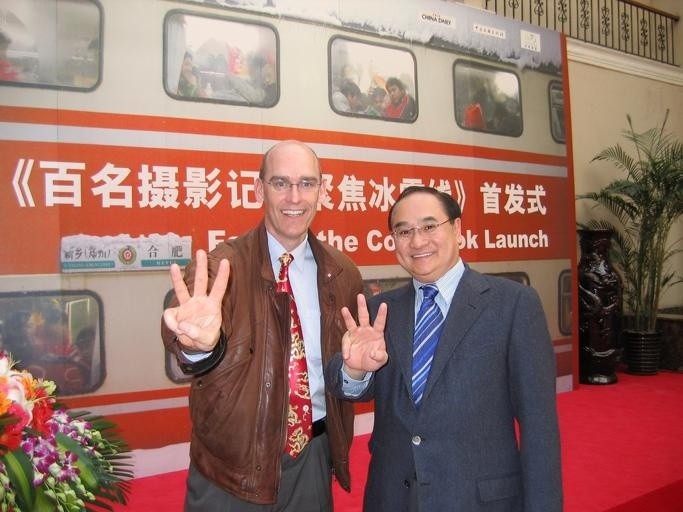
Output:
[412,285,446,410]
[278,253,314,459]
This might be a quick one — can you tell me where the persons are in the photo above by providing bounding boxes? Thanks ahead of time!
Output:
[178,53,200,96]
[386,78,415,119]
[230,62,277,106]
[324,186,563,512]
[1,309,95,391]
[366,88,386,117]
[161,140,365,512]
[332,81,361,113]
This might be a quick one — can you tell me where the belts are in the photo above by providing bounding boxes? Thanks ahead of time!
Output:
[312,417,327,439]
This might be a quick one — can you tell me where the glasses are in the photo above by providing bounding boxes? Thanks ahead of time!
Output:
[262,176,320,193]
[390,220,451,241]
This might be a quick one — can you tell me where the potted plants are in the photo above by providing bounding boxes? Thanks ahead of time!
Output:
[572,103,682,375]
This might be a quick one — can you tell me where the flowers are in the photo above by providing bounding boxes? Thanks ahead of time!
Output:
[1,350,140,512]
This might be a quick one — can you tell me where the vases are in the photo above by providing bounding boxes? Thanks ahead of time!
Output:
[576,226,624,386]
